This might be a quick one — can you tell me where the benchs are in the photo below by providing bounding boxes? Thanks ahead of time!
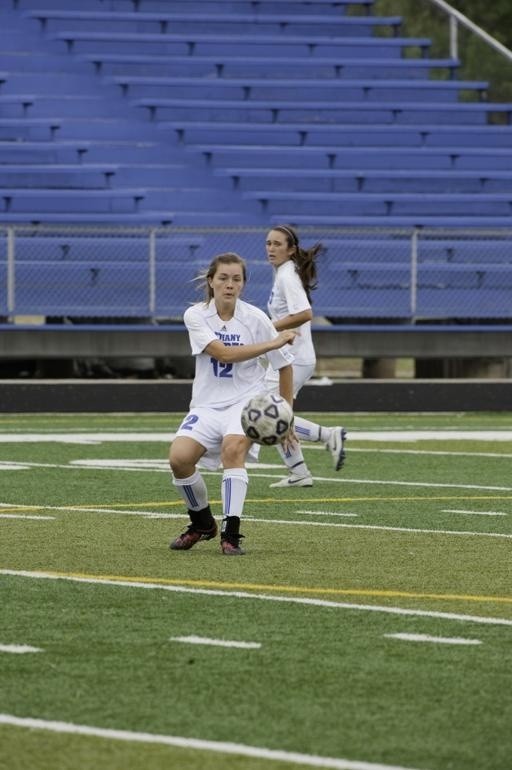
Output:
[0,10,512,318]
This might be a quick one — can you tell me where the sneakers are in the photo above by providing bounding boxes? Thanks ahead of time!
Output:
[220,532,245,555]
[170,518,218,550]
[268,472,313,488]
[325,426,347,471]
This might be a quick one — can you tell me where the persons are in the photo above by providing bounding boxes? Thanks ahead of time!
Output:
[264,225,348,490]
[167,251,302,557]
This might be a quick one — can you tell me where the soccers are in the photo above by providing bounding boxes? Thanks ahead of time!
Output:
[241,392,293,447]
